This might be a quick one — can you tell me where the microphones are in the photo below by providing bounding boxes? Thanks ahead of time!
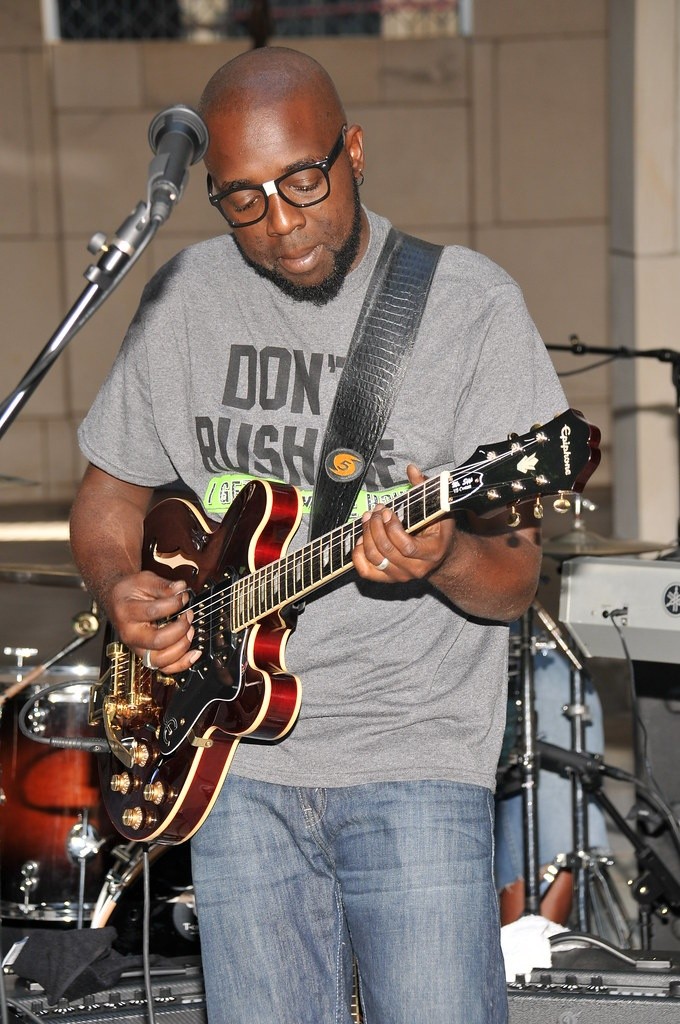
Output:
[148,102,208,223]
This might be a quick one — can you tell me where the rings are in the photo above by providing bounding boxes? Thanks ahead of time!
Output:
[139,648,160,672]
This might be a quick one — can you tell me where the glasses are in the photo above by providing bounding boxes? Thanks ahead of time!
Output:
[206,123,348,227]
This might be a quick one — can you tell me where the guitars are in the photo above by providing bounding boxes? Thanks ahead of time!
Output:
[96,408,603,847]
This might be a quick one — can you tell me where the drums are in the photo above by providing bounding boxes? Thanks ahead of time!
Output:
[3,667,118,922]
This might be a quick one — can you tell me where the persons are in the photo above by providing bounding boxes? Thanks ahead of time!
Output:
[67,47,576,1024]
